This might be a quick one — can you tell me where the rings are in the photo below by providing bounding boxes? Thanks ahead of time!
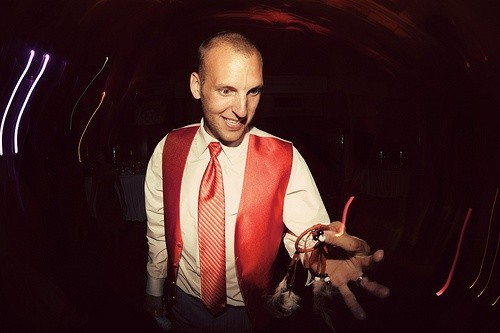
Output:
[356,274,367,284]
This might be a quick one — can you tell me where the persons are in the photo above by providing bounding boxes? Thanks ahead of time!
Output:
[144,30,389,333]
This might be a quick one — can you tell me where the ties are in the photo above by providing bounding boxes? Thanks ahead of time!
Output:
[197,141,227,319]
